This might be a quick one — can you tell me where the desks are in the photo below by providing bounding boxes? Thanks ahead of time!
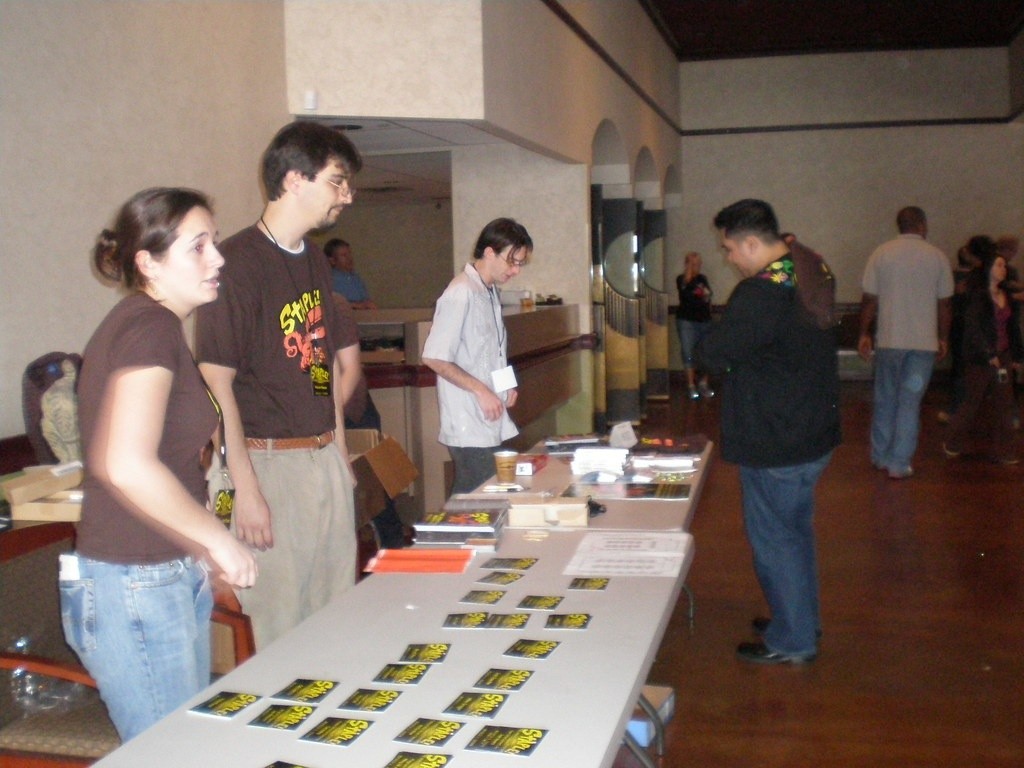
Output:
[89,527,697,768]
[442,434,715,768]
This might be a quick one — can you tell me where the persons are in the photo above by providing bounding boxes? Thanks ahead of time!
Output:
[940,235,1024,465]
[324,238,378,309]
[675,252,715,399]
[781,233,837,349]
[421,217,534,497]
[858,206,954,479]
[693,198,840,665]
[59,188,258,744]
[195,120,365,654]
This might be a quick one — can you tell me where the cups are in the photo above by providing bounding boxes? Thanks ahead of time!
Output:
[494,451,518,484]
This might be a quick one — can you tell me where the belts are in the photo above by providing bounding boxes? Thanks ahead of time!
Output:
[242,430,336,451]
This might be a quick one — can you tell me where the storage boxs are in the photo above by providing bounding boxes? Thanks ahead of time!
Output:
[620,685,675,750]
[345,429,421,533]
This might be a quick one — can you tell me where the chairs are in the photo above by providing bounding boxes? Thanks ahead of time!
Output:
[0,520,258,768]
[21,352,85,466]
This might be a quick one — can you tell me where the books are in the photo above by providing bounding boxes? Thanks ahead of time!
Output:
[544,438,702,501]
[412,492,591,544]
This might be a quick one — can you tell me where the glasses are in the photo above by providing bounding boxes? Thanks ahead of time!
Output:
[312,172,358,200]
[498,253,528,268]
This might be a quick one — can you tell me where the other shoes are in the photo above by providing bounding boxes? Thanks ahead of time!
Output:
[737,616,823,663]
[687,382,716,399]
[876,459,916,479]
